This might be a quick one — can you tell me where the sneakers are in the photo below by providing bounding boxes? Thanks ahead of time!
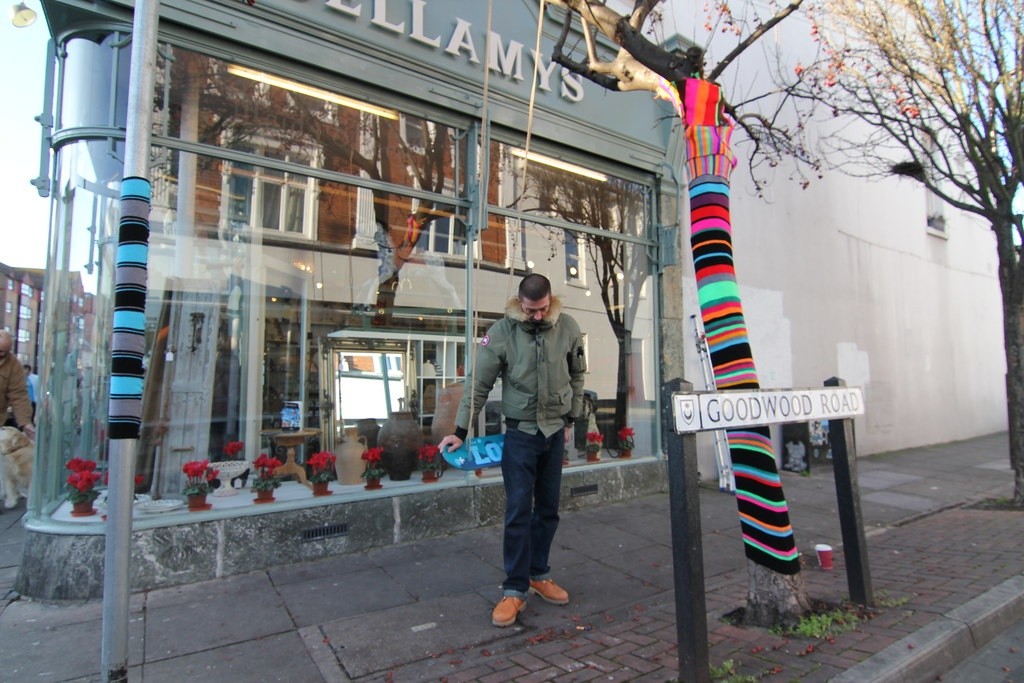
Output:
[530,579,570,605]
[492,596,527,627]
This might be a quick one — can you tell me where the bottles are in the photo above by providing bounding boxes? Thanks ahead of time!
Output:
[376,411,423,481]
[356,418,381,449]
[334,428,369,485]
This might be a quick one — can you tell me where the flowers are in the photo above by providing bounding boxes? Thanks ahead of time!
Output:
[583,431,604,451]
[617,427,634,450]
[223,441,245,461]
[63,457,101,504]
[360,447,384,479]
[177,461,220,500]
[415,444,441,472]
[303,450,335,485]
[249,454,282,493]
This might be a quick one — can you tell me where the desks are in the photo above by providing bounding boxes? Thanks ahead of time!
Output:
[257,427,321,488]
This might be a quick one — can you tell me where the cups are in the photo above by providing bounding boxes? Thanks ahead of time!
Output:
[815,543,834,570]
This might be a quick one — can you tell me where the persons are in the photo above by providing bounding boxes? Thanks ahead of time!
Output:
[438,273,587,625]
[0,329,39,440]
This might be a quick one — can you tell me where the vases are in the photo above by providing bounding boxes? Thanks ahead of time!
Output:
[72,503,93,513]
[422,470,435,479]
[187,492,205,509]
[312,482,328,494]
[334,429,370,481]
[585,451,596,461]
[209,461,249,497]
[255,489,273,500]
[619,449,630,457]
[432,382,467,447]
[366,476,379,486]
[378,408,420,483]
